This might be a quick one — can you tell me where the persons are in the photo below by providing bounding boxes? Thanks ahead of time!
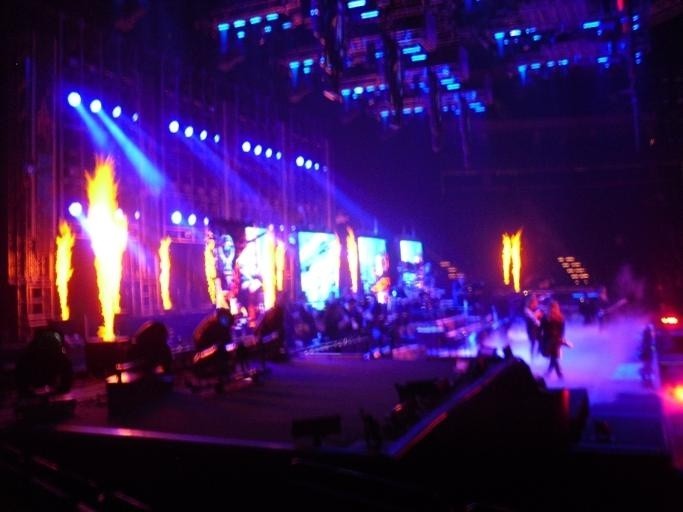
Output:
[192,293,409,376]
[522,294,570,383]
[15,329,73,401]
[128,318,173,371]
